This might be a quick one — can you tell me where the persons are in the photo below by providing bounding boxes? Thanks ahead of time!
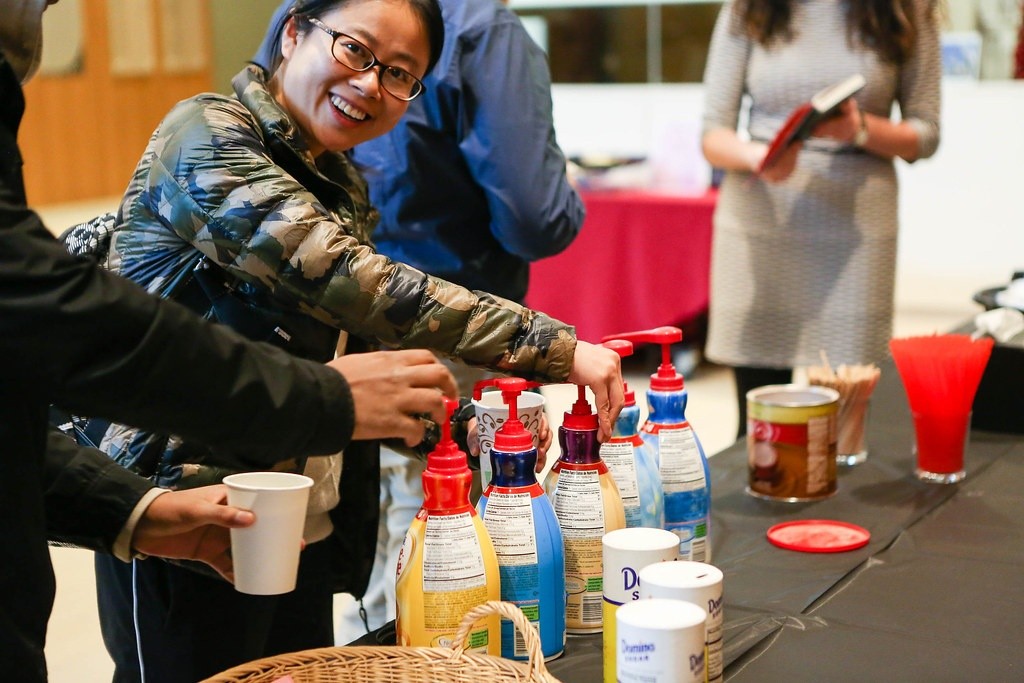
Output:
[93,0,625,683]
[252,0,586,648]
[701,0,943,442]
[0,0,459,683]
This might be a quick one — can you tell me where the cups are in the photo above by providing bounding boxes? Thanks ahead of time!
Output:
[887,307,994,484]
[471,391,546,493]
[837,401,872,466]
[221,472,315,596]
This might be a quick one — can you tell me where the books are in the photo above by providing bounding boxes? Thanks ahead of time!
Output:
[756,74,868,176]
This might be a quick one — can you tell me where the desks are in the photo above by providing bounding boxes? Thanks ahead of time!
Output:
[339,323,1024,683]
[523,182,720,366]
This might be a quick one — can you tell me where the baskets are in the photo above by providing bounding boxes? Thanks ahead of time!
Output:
[201,600,561,683]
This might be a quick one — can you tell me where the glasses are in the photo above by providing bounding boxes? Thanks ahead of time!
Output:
[305,13,427,101]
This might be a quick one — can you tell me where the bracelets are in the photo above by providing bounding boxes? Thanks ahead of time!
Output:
[854,110,869,147]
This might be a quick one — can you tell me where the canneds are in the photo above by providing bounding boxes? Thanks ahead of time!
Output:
[746,384,842,502]
